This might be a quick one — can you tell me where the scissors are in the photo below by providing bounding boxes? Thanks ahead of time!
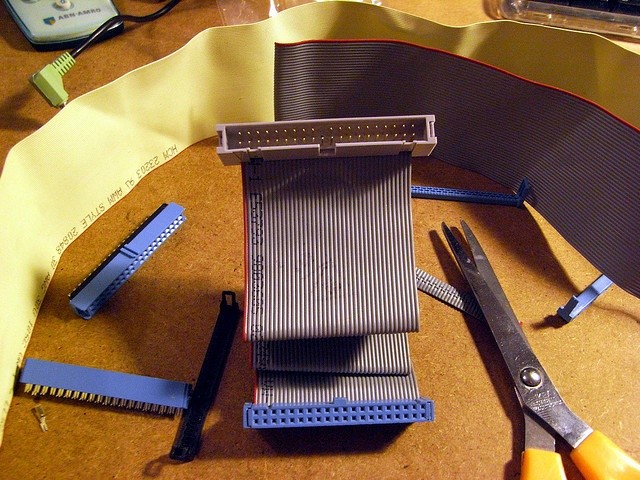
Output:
[442,216,640,480]
